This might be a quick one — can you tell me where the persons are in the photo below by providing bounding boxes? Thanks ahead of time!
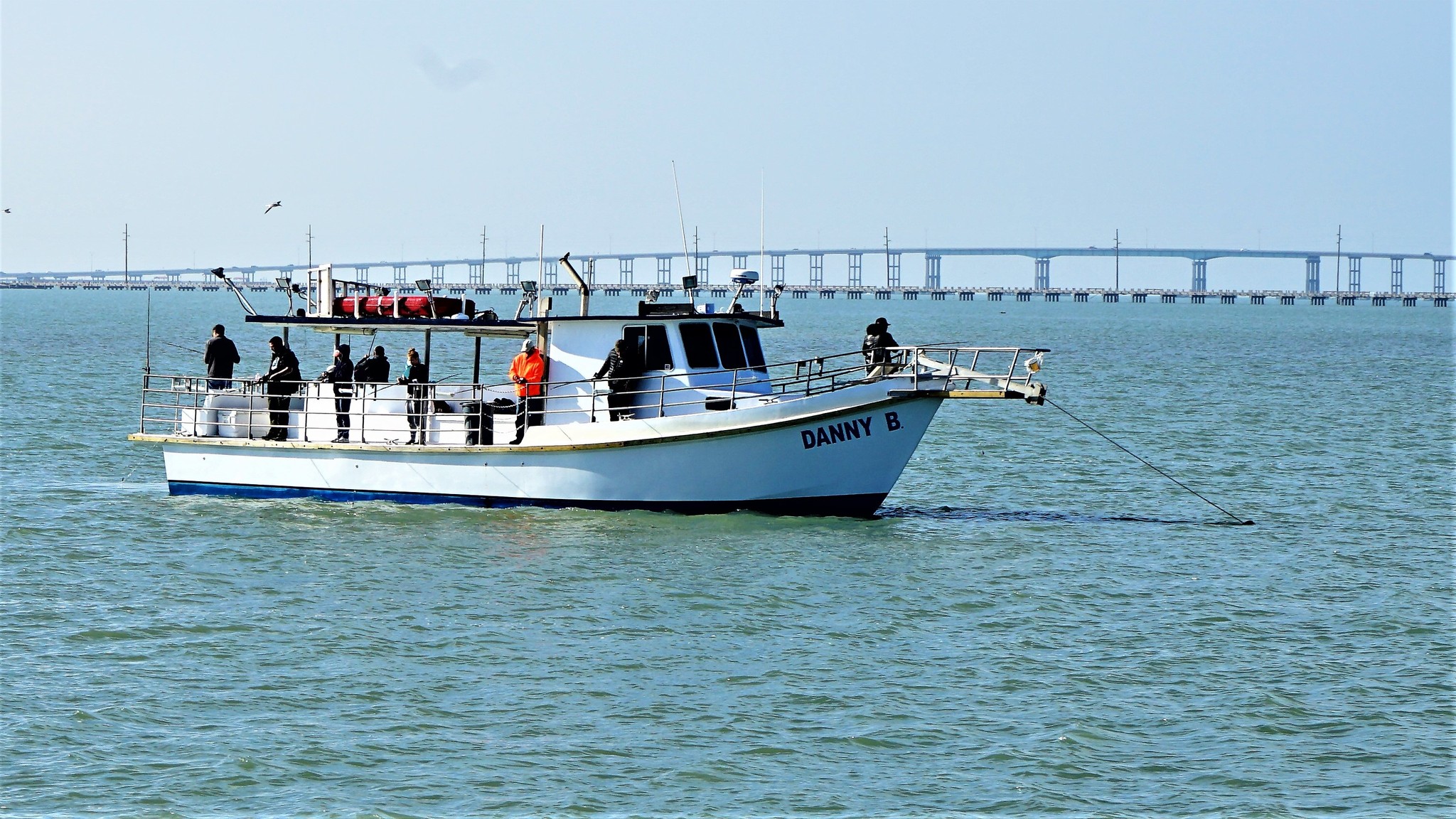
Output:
[862,318,900,377]
[322,344,354,443]
[594,339,639,421]
[508,339,544,444]
[353,346,390,382]
[258,336,302,442]
[396,347,429,445]
[204,324,240,389]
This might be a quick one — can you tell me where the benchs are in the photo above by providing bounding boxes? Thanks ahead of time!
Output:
[182,394,303,437]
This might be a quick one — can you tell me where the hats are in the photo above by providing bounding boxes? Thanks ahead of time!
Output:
[521,340,534,351]
[876,317,891,326]
[615,339,625,351]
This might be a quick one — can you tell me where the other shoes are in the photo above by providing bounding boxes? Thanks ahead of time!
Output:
[331,437,339,442]
[413,441,426,445]
[509,439,521,444]
[274,437,286,441]
[261,434,276,440]
[338,436,349,442]
[405,440,415,444]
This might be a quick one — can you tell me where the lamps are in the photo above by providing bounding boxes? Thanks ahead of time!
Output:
[276,277,291,288]
[683,275,697,289]
[521,280,537,292]
[376,288,391,296]
[415,279,432,291]
[211,267,225,278]
[774,283,786,295]
[646,288,660,302]
[292,283,300,293]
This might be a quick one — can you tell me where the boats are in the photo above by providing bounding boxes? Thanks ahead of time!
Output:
[128,158,1052,514]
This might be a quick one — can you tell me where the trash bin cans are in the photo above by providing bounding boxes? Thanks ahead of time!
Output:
[459,400,497,445]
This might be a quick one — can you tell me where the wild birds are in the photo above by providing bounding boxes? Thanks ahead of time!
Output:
[264,201,282,215]
[1,208,12,214]
